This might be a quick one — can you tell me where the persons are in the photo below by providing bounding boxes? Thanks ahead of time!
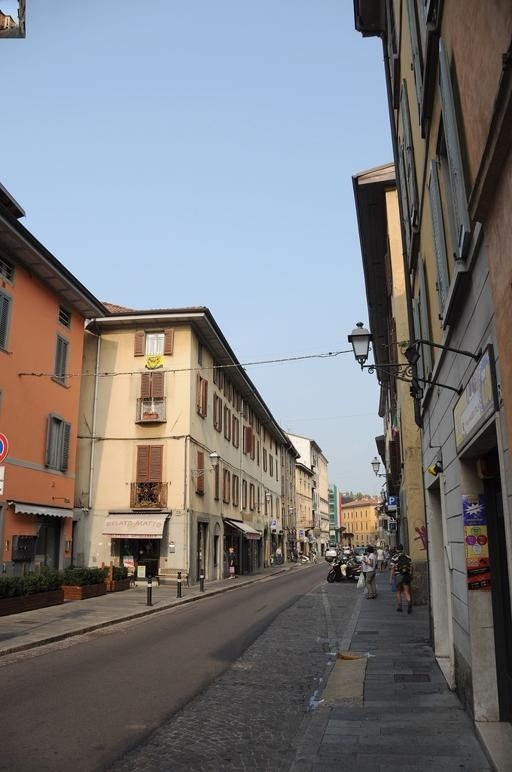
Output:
[365,548,378,601]
[361,552,367,582]
[275,544,283,564]
[380,539,414,616]
[227,547,239,581]
[312,551,318,566]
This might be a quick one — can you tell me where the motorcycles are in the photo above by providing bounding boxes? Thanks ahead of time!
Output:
[299,550,319,565]
[326,558,365,584]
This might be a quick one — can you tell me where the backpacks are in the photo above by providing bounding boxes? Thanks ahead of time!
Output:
[397,552,410,575]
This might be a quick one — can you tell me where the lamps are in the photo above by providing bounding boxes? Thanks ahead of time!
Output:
[52,495,72,504]
[402,337,482,365]
[371,456,400,478]
[191,450,220,479]
[348,321,417,384]
[257,492,271,506]
[408,376,464,401]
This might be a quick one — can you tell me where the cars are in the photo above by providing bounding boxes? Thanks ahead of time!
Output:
[324,545,370,563]
